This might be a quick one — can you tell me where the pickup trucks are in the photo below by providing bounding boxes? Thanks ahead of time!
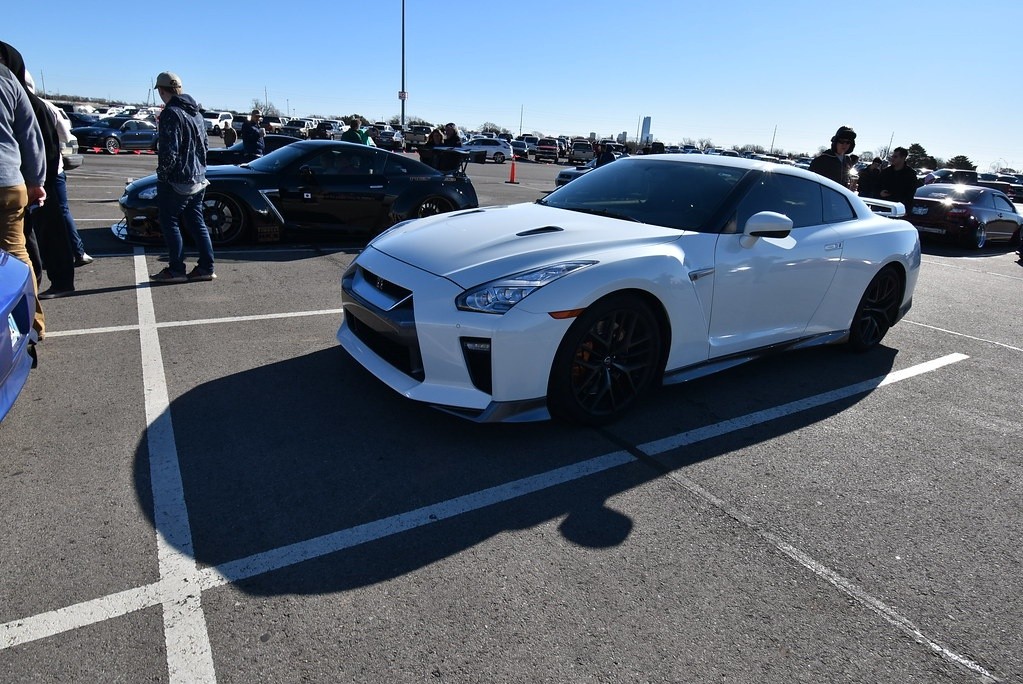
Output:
[404,125,436,152]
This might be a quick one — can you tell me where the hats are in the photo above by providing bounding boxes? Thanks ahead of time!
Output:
[251,110,262,117]
[831,127,856,155]
[224,121,230,129]
[153,71,181,89]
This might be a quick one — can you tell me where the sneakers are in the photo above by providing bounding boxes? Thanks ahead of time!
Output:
[149,267,185,281]
[186,266,216,280]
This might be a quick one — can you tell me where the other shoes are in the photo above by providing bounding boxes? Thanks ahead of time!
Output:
[37,285,73,299]
[75,253,94,265]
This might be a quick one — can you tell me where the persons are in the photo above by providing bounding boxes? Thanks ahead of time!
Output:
[596,145,616,166]
[220,122,237,147]
[149,73,217,282]
[917,174,936,188]
[424,123,462,148]
[808,127,917,219]
[342,119,379,145]
[242,110,265,162]
[0,40,94,341]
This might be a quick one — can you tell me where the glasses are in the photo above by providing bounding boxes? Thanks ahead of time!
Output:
[837,140,851,144]
[891,155,898,158]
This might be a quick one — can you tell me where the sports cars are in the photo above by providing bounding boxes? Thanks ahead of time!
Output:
[111,139,479,251]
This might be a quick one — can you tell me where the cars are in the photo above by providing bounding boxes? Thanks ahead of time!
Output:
[336,154,921,427]
[0,248,39,424]
[465,133,874,192]
[913,168,1023,203]
[69,117,159,155]
[61,103,350,140]
[433,138,514,164]
[911,184,1023,255]
[360,122,409,152]
